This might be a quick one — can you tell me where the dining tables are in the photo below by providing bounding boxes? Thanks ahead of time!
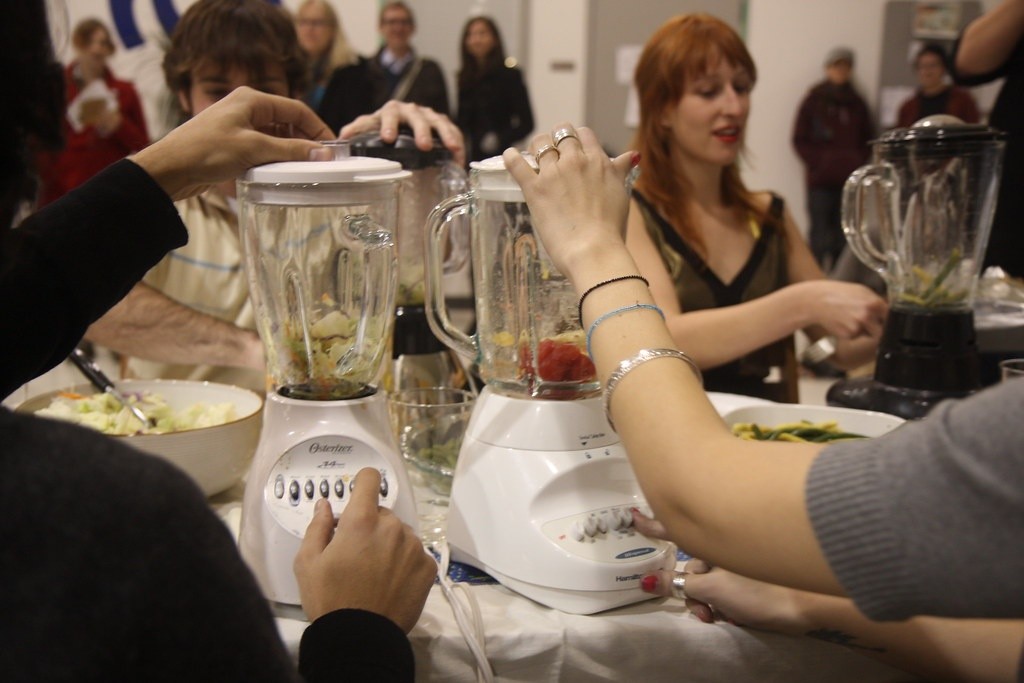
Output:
[207,355,934,682]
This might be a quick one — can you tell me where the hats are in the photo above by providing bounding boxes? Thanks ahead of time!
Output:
[825,47,852,66]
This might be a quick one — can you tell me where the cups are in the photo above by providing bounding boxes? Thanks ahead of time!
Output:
[1001,360,1023,385]
[385,388,478,544]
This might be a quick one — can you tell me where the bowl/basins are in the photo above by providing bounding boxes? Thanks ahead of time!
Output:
[400,411,475,496]
[14,380,264,497]
[720,404,905,445]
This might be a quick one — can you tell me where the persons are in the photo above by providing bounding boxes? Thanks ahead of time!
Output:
[0,86,438,683]
[0,0,269,370]
[898,45,981,126]
[624,14,890,405]
[946,0,1024,276]
[456,16,534,162]
[791,48,875,271]
[35,21,150,211]
[367,2,449,116]
[128,0,462,400]
[502,122,1024,683]
[281,0,380,135]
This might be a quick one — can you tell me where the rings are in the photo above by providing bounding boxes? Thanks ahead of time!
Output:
[535,144,560,165]
[553,128,579,147]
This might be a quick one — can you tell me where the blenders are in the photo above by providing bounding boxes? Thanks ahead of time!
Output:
[345,135,470,430]
[236,141,423,605]
[424,153,678,615]
[841,114,1010,418]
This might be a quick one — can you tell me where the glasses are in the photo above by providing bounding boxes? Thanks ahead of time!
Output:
[383,17,411,25]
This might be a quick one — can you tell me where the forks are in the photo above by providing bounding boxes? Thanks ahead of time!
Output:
[801,334,839,366]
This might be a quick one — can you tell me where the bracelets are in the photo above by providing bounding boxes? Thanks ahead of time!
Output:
[585,304,666,362]
[601,348,703,432]
[577,275,650,328]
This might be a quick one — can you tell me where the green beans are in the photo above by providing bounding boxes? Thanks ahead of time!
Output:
[751,424,867,442]
